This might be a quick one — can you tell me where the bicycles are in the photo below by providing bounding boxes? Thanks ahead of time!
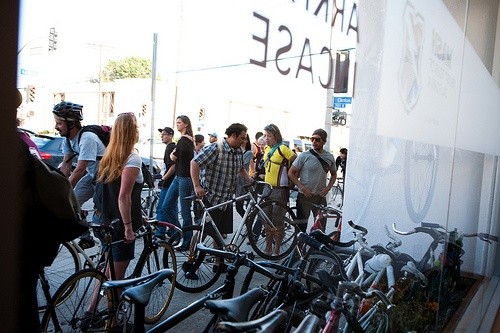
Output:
[162,177,299,294]
[139,187,160,231]
[324,176,343,214]
[38,219,500,333]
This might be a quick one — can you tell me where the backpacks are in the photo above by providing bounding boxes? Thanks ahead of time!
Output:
[81,125,111,162]
[16,136,89,271]
[288,160,300,190]
[256,152,265,175]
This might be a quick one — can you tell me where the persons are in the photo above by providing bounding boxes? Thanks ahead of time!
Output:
[15,88,42,333]
[254,124,301,258]
[284,144,302,155]
[182,123,255,273]
[288,128,337,260]
[234,132,267,245]
[52,102,106,253]
[336,148,348,178]
[80,113,145,333]
[151,115,218,251]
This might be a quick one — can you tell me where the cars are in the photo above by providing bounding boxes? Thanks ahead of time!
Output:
[21,128,162,183]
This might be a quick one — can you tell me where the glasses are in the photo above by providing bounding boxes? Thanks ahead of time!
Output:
[310,137,321,143]
[118,113,134,116]
[159,134,167,136]
[264,126,276,132]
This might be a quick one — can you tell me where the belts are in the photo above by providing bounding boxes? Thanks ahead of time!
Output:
[94,208,103,217]
[269,186,289,189]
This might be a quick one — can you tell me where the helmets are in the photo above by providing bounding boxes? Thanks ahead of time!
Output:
[52,100,84,123]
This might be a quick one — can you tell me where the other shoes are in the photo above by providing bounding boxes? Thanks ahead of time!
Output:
[253,250,273,258]
[72,237,95,253]
[172,240,180,246]
[165,233,171,243]
[181,260,192,273]
[81,210,89,219]
[176,245,190,251]
[81,310,104,330]
[271,253,279,257]
[152,237,165,243]
[212,264,229,273]
[108,321,133,333]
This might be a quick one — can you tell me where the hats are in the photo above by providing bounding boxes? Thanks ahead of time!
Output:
[208,132,217,138]
[157,126,174,135]
[312,128,327,141]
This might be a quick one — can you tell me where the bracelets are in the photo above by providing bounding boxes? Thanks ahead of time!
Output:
[124,221,132,225]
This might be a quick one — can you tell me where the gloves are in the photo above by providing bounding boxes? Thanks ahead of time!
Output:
[158,178,166,189]
